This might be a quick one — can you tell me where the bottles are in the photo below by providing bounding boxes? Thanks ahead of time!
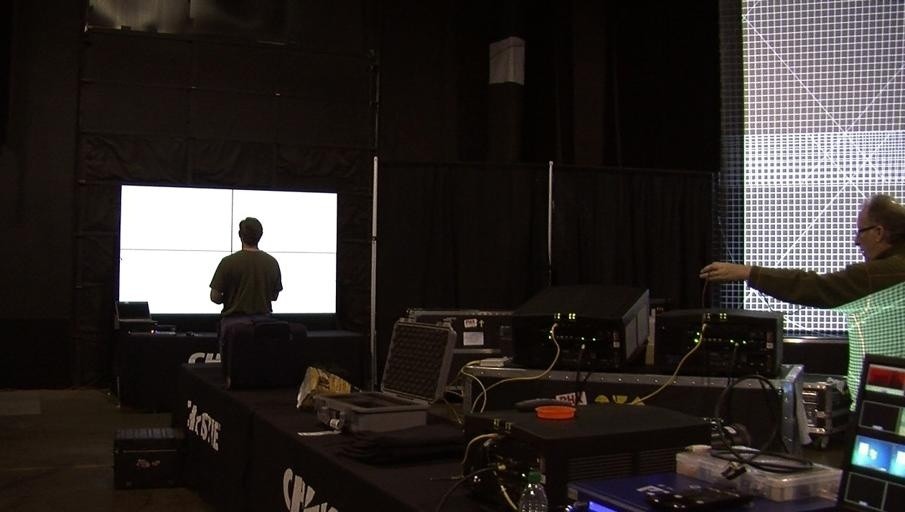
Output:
[517,466,549,512]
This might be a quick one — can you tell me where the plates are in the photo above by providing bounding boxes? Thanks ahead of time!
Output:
[535,402,576,419]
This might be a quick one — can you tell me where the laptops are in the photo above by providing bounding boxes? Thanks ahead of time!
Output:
[116,302,160,323]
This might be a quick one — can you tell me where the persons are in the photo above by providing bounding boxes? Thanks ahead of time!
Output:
[210,219,283,347]
[700,194,905,415]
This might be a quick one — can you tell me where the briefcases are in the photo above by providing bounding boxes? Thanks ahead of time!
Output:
[313,318,457,434]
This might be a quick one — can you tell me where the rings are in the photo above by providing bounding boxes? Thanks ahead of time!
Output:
[706,271,710,276]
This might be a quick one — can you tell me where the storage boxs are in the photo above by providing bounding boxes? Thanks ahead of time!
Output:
[112,427,189,491]
